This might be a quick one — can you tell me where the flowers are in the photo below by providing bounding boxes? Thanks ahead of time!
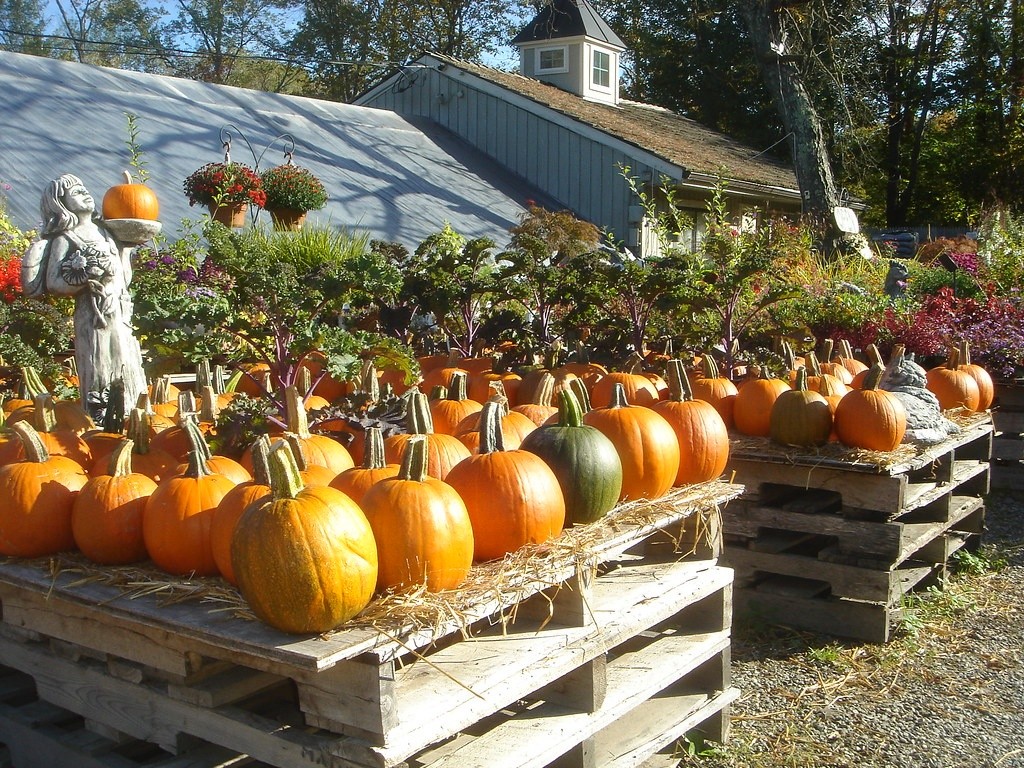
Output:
[184,162,267,208]
[260,164,328,211]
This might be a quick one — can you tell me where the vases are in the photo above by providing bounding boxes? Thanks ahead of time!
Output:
[207,202,247,228]
[269,210,308,230]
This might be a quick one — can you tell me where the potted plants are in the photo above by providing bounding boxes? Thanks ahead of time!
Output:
[660,208,694,242]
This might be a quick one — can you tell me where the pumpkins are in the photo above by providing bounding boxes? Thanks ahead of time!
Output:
[690,338,995,452]
[102,170,158,221]
[0,348,728,633]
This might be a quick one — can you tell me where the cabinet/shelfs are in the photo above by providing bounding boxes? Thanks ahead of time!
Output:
[721,410,996,643]
[1,481,744,768]
[990,377,1024,493]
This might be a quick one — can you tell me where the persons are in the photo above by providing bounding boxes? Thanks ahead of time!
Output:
[41,174,155,422]
